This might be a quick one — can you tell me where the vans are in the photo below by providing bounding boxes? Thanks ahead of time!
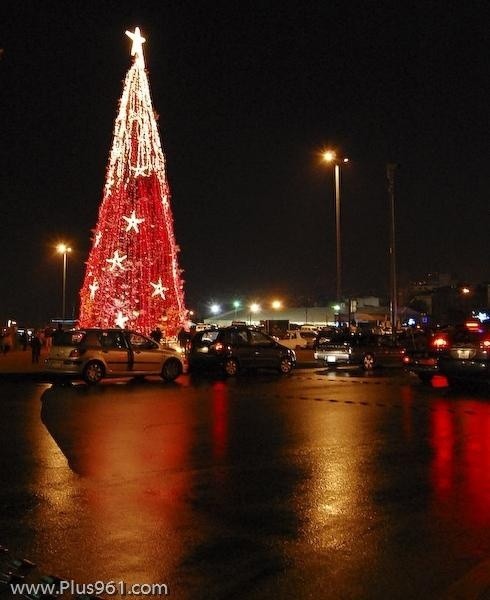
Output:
[43,329,188,385]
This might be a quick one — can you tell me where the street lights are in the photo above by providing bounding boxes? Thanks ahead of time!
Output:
[248,302,260,325]
[322,148,350,305]
[56,243,73,319]
[233,302,240,320]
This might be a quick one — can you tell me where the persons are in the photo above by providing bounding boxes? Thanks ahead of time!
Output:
[30,337,42,363]
[175,327,187,349]
[0,323,66,360]
